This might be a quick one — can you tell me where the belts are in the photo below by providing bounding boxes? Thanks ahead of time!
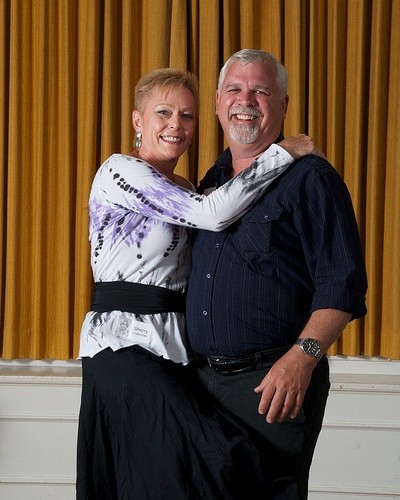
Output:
[195,352,281,375]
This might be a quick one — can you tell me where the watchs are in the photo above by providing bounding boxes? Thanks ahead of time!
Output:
[294,338,323,362]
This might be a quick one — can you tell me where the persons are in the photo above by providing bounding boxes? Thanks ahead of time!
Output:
[184,49,368,500]
[76,68,327,500]
